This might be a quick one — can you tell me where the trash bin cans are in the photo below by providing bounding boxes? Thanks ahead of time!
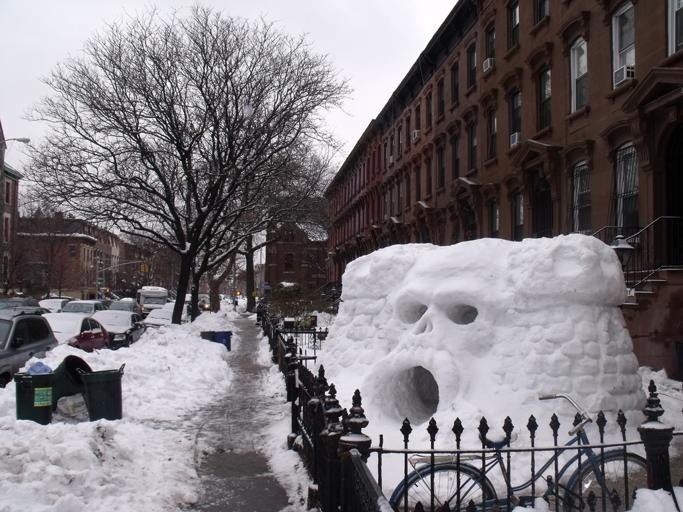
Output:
[202,331,233,351]
[14,355,125,425]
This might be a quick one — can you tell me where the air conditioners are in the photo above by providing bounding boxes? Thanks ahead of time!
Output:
[614,64,635,86]
[483,57,495,72]
[510,132,521,147]
[413,129,420,139]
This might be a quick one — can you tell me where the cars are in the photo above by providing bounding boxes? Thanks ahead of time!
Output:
[0,283,230,388]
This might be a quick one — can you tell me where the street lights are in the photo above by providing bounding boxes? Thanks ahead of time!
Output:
[0,136,31,147]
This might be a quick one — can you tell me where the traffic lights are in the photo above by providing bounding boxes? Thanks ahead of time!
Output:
[140,263,144,272]
[234,290,241,295]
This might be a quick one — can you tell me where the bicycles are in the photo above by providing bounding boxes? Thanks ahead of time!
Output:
[383,388,650,511]
